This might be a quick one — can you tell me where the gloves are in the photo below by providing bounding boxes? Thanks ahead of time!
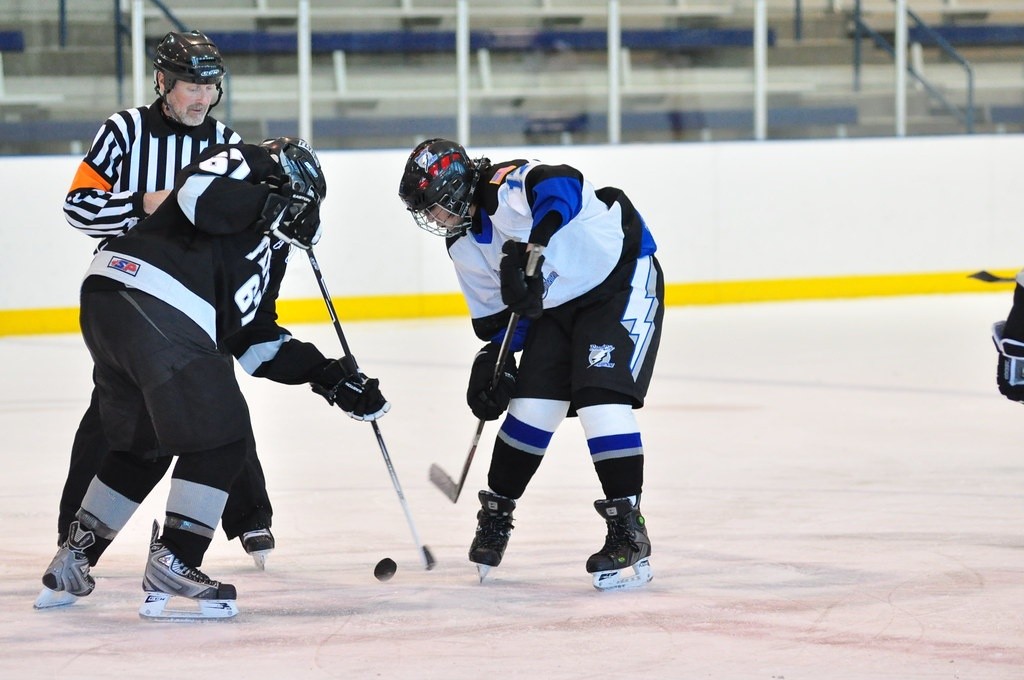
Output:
[263,190,320,247]
[312,355,392,420]
[499,239,545,318]
[466,341,518,420]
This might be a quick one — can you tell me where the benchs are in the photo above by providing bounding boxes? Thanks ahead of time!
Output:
[907,22,1024,94]
[0,105,859,157]
[201,28,787,100]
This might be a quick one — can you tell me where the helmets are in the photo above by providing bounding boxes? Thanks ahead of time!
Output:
[399,138,478,213]
[257,137,327,202]
[153,30,226,91]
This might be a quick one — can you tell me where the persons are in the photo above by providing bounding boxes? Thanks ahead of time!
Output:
[398,137,668,590]
[989,266,1024,404]
[56,30,275,570]
[32,136,390,619]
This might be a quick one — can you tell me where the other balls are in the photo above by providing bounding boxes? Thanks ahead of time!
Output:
[374,558,398,582]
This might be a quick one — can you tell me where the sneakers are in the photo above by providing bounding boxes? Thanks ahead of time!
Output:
[467,490,518,584]
[35,523,95,608]
[140,522,240,621]
[586,493,652,592]
[239,529,276,570]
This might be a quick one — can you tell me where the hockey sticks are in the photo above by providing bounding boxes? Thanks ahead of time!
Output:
[301,243,438,575]
[427,246,542,505]
